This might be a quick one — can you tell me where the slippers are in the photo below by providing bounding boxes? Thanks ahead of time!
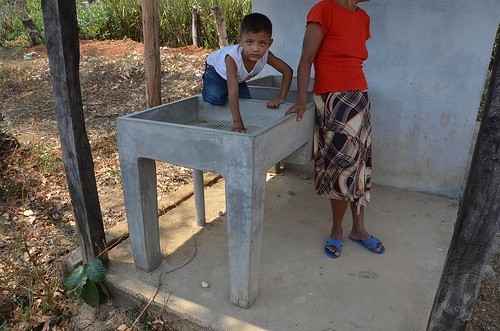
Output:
[349,235,385,254]
[325,236,343,258]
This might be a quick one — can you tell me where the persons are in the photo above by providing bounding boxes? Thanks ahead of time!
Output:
[201,13,294,134]
[284,0,386,256]
